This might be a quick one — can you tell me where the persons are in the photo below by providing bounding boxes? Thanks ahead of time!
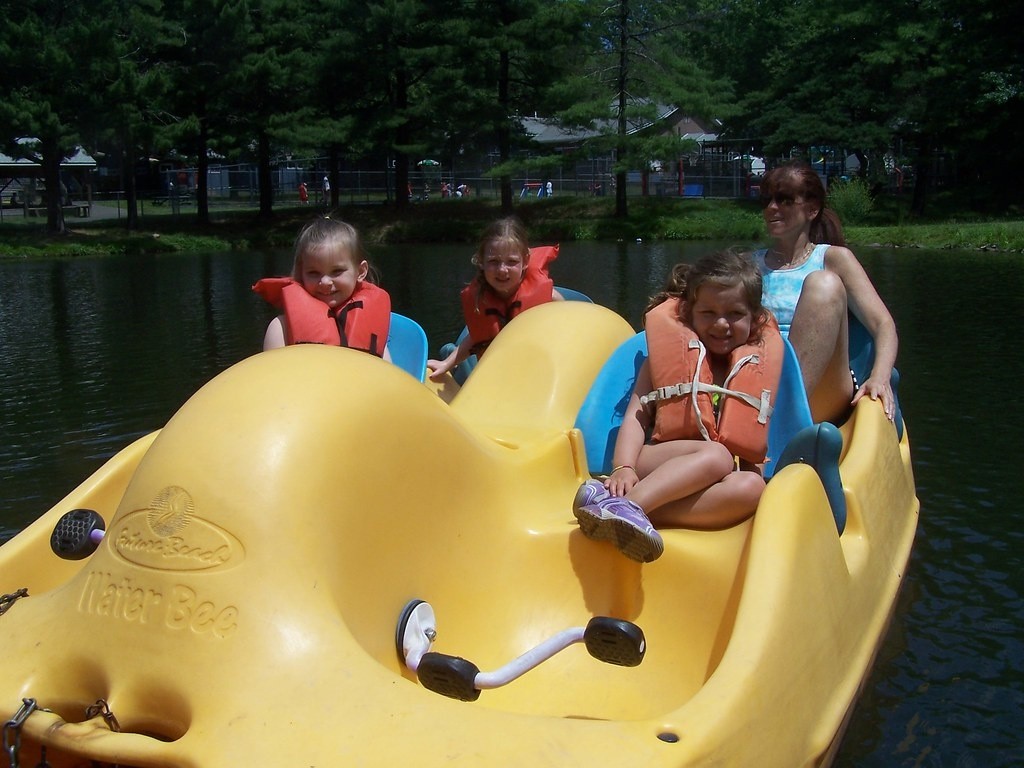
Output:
[587,175,617,197]
[407,181,470,201]
[299,182,309,206]
[571,248,785,562]
[426,218,565,379]
[545,179,553,199]
[250,210,392,365]
[10,192,18,205]
[317,176,330,207]
[738,160,898,424]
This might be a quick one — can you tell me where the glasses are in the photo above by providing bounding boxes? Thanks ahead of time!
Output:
[759,191,806,210]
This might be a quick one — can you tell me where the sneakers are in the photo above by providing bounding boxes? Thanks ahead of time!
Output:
[578,498,664,562]
[573,479,613,518]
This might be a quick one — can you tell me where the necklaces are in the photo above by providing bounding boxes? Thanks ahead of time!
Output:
[771,242,813,266]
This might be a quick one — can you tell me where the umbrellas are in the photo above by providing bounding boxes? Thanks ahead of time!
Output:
[417,159,441,167]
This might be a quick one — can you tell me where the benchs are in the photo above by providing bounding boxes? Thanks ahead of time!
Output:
[151,195,193,207]
[0,201,91,217]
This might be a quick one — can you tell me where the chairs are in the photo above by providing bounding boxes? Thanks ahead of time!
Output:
[384,312,428,387]
[439,287,595,387]
[563,330,848,541]
[844,319,906,444]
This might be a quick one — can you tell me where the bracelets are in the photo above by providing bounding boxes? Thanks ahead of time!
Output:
[611,465,636,476]
